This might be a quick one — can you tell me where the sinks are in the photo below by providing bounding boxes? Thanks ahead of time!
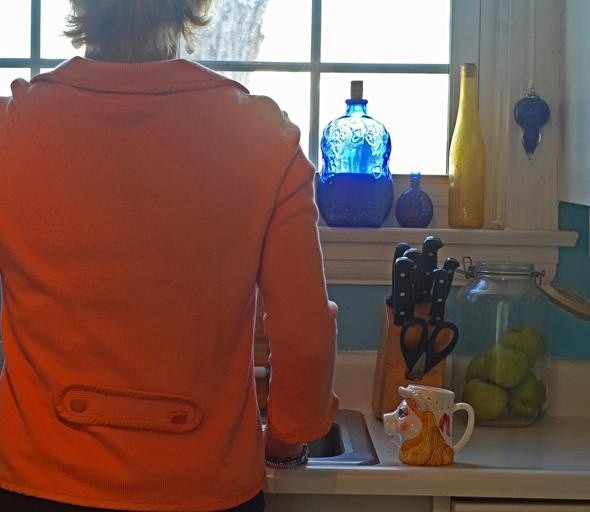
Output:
[262,408,380,467]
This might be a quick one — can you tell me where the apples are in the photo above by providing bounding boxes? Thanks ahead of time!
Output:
[461,325,547,425]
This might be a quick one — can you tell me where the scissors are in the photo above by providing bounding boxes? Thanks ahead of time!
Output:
[400,318,459,380]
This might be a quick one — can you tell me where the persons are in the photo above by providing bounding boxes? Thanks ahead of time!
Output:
[0,1,340,512]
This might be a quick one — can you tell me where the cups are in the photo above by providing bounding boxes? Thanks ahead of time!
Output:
[383,385,480,465]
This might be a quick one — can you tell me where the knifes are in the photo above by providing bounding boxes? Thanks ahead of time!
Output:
[389,236,456,319]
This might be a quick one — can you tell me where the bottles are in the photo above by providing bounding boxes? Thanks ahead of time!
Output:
[395,172,436,227]
[312,80,395,225]
[445,65,485,231]
[455,260,552,430]
